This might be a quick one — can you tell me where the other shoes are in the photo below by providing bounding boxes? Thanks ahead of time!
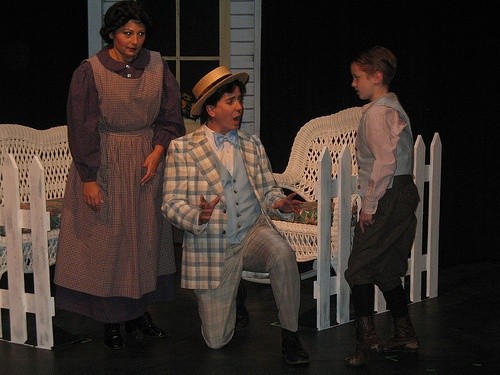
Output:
[101,323,125,349]
[125,316,166,339]
[281,330,309,364]
[236,282,249,329]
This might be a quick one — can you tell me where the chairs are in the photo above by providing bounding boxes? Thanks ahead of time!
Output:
[240,106,369,283]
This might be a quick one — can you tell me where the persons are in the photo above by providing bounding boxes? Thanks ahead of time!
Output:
[161,65,310,367]
[344,46,420,367]
[54,0,187,350]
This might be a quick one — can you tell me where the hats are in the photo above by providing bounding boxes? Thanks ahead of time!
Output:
[191,65,249,115]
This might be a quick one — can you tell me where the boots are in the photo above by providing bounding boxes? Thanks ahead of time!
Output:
[382,313,419,354]
[345,315,384,366]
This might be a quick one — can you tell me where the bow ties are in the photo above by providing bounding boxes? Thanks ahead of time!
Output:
[213,130,240,148]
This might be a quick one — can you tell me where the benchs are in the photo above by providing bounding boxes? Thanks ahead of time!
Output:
[0,118,200,279]
